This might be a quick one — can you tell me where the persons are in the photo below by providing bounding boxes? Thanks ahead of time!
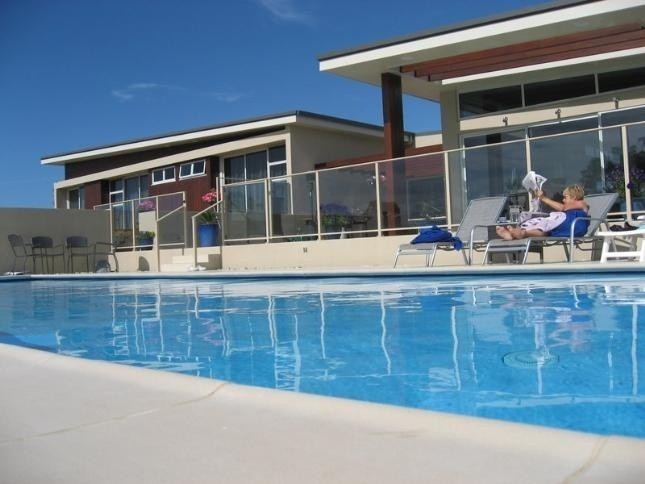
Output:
[496,183,589,239]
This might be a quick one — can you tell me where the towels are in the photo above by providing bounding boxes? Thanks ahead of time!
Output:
[547,208,591,238]
[409,223,464,252]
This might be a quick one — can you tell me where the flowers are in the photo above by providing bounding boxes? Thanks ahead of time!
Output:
[321,204,352,226]
[135,200,155,211]
[200,191,219,206]
[602,165,645,198]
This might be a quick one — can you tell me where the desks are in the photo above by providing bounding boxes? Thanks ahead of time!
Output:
[593,227,645,264]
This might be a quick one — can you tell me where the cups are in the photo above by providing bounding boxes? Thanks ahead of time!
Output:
[509,204,523,222]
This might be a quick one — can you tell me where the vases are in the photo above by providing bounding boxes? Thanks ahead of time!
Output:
[325,225,340,239]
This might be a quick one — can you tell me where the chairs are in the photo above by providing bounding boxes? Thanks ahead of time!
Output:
[6,233,120,277]
[481,192,621,264]
[392,196,508,267]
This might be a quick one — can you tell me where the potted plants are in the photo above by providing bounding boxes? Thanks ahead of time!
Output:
[194,210,219,247]
[621,197,644,219]
[135,229,156,250]
